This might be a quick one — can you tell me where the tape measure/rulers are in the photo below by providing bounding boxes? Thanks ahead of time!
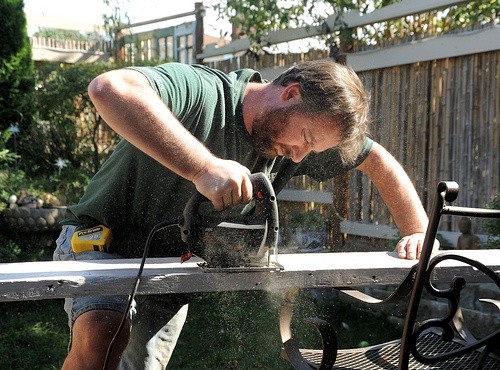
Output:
[70,223,114,253]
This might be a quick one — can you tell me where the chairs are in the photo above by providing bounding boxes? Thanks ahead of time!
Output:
[280,181,500,370]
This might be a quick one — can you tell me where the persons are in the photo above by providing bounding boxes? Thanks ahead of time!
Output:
[49,59,444,369]
